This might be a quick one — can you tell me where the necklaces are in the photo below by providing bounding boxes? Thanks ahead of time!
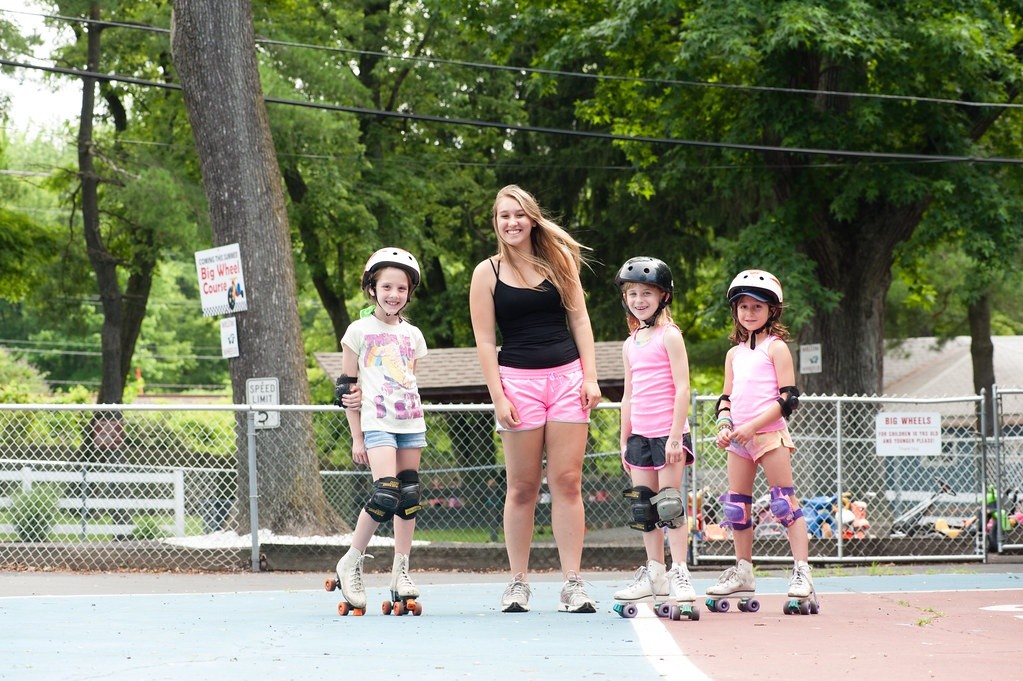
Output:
[636,337,651,345]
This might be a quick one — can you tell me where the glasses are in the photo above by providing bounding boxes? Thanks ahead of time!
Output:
[859,508,864,512]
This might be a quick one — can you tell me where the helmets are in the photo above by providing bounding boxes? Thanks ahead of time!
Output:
[362,247,420,290]
[615,256,673,304]
[727,269,783,318]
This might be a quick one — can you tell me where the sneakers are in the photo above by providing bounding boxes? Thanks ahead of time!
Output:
[558,570,597,613]
[501,572,533,612]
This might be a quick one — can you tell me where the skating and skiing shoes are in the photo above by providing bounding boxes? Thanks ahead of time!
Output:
[613,559,670,618]
[382,553,422,616]
[783,559,820,615]
[705,558,760,612]
[665,563,700,621]
[324,545,374,616]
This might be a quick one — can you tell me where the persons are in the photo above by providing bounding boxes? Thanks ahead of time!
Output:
[614,256,695,602]
[334,247,429,609]
[706,270,814,597]
[469,185,602,613]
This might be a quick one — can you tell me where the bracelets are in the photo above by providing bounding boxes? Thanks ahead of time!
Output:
[583,379,597,383]
[717,417,733,433]
